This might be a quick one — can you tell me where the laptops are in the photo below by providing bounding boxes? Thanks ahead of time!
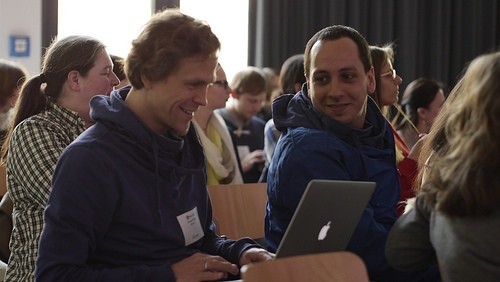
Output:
[274,179,377,258]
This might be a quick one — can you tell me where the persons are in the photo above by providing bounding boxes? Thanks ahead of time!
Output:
[0,36,131,282]
[34,9,276,282]
[192,25,500,282]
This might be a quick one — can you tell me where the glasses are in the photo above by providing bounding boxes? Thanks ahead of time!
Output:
[215,80,228,88]
[378,69,397,79]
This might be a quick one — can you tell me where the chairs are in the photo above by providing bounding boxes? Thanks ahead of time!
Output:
[204,183,267,241]
[238,251,370,282]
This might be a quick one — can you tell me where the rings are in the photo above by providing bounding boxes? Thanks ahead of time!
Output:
[204,260,208,271]
